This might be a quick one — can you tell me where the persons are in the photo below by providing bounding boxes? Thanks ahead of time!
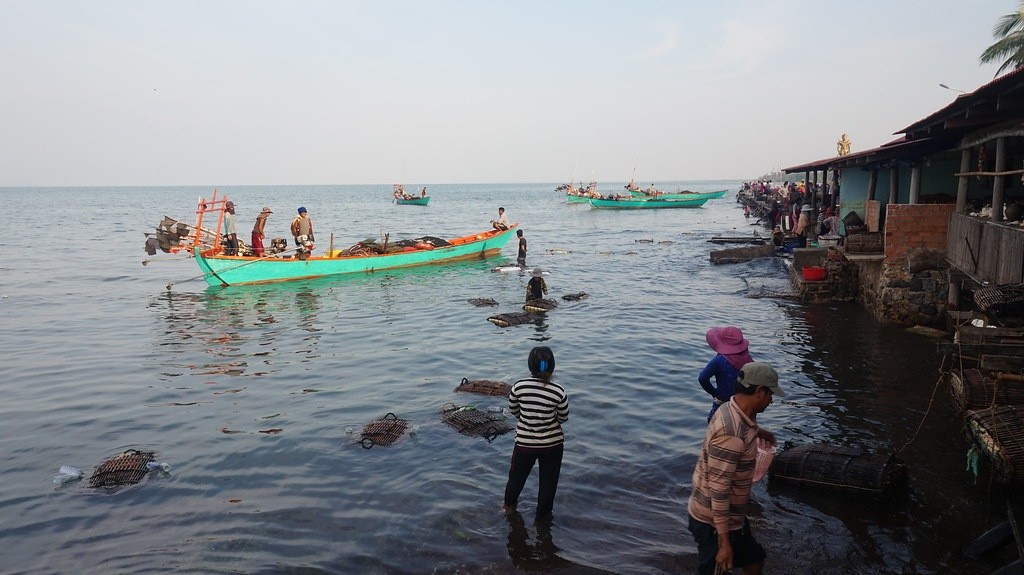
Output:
[291,207,315,245]
[741,179,839,247]
[688,361,787,575]
[696,326,754,425]
[837,133,851,156]
[504,345,570,514]
[394,186,427,200]
[224,201,239,257]
[252,207,273,258]
[516,229,527,268]
[489,207,510,233]
[556,183,654,199]
[525,268,547,301]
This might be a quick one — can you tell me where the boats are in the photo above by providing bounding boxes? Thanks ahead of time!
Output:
[394,185,431,206]
[191,187,521,286]
[589,197,707,209]
[565,183,729,203]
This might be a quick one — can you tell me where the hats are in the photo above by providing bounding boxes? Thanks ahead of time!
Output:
[225,200,237,210]
[819,206,827,211]
[737,362,785,397]
[261,207,273,214]
[834,208,839,213]
[706,326,749,355]
[826,207,834,213]
[529,268,544,277]
[773,227,781,233]
[297,207,307,214]
[801,204,814,211]
